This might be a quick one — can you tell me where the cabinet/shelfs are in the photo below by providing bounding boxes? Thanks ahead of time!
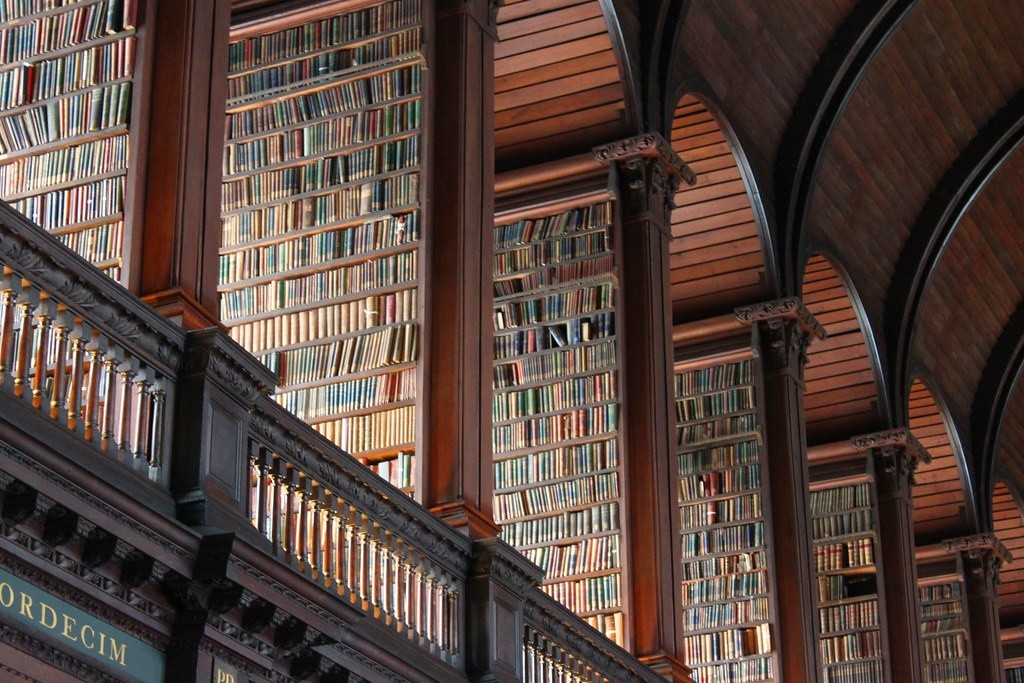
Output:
[916,574,975,683]
[673,347,784,683]
[0,0,157,444]
[807,471,891,683]
[215,0,436,620]
[489,189,634,683]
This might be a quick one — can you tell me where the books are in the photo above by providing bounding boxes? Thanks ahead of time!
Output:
[2,0,137,451]
[807,483,886,683]
[213,1,430,611]
[493,202,631,682]
[917,576,968,681]
[1007,664,1024,683]
[671,355,784,683]
